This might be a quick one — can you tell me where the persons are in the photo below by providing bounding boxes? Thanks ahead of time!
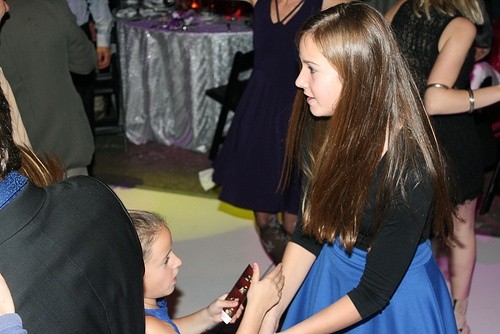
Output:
[0,0,500,334]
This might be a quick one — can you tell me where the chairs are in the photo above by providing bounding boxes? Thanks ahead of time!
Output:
[204,49,255,159]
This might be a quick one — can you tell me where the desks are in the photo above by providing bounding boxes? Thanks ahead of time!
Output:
[112,15,255,152]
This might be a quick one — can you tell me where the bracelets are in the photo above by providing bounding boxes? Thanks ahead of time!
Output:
[425,82,450,90]
[467,87,474,114]
